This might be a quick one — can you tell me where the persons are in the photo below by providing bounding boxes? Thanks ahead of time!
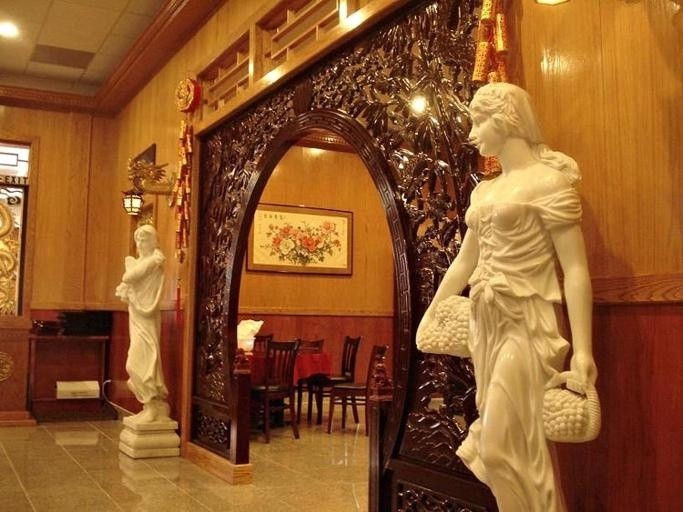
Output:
[431,83,599,505]
[111,223,173,422]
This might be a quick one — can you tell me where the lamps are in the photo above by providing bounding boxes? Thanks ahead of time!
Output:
[120,182,144,216]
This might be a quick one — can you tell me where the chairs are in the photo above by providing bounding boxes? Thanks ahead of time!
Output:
[251,331,386,445]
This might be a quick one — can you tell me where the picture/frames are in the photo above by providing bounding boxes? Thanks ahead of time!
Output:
[246,202,353,275]
